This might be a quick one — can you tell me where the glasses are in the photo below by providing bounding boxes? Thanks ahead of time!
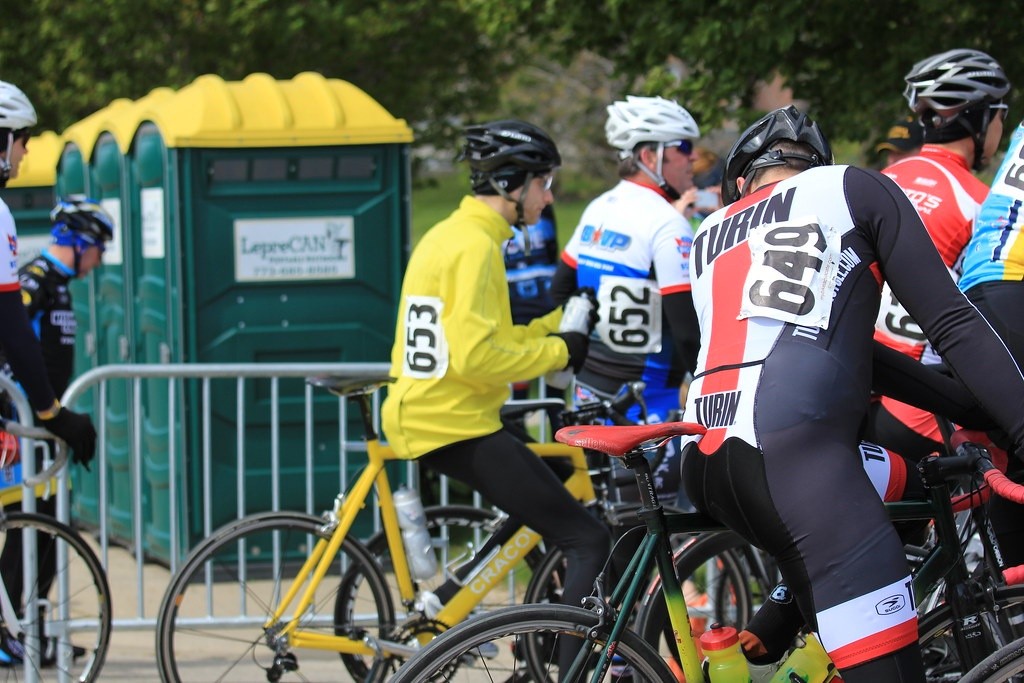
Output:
[664,139,693,155]
[965,105,1009,122]
[533,173,553,191]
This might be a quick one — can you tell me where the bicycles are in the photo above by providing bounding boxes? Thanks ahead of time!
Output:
[380,421,1024,683]
[154,368,783,683]
[0,355,112,683]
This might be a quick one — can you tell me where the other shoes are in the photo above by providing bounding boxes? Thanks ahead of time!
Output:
[0,628,85,668]
[611,655,633,683]
[701,646,796,683]
[414,590,498,657]
[511,634,600,669]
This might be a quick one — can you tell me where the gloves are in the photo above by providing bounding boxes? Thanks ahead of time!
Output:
[41,407,96,472]
[547,333,589,374]
[562,286,600,335]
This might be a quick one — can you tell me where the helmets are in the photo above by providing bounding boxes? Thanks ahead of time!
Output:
[0,80,37,131]
[604,95,701,150]
[722,105,832,207]
[903,48,1011,130]
[462,120,562,195]
[50,194,114,241]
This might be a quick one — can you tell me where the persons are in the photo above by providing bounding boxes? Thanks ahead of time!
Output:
[867,48,1024,584]
[0,203,115,667]
[380,120,613,683]
[506,94,724,683]
[0,74,98,474]
[677,148,731,215]
[678,105,1024,683]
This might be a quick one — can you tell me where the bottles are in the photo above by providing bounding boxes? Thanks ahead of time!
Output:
[546,291,595,391]
[393,482,439,582]
[699,623,752,683]
[769,632,835,683]
